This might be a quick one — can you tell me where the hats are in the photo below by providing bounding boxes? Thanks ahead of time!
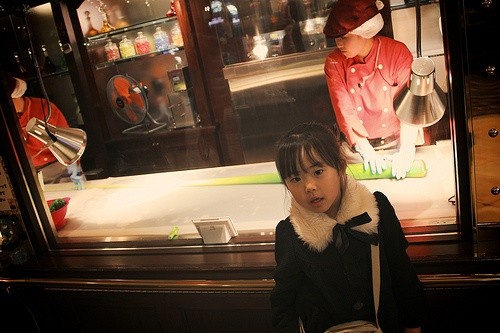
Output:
[322,0,379,38]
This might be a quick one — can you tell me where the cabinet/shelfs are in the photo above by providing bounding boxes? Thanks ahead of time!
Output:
[0,0,245,172]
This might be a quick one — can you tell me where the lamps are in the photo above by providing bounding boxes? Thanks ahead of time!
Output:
[393,0,447,128]
[22,14,88,166]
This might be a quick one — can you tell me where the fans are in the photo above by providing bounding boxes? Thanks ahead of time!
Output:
[106,74,167,135]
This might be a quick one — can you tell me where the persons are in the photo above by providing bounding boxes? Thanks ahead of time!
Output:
[10,78,87,185]
[270,121,426,333]
[323,0,438,180]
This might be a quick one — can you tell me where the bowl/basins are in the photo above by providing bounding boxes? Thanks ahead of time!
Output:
[47,197,71,229]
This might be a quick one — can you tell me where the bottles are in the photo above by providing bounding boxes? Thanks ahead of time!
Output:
[153,27,170,51]
[166,1,177,17]
[98,6,115,33]
[134,32,151,55]
[85,11,99,37]
[104,39,120,62]
[119,35,136,59]
[172,23,184,47]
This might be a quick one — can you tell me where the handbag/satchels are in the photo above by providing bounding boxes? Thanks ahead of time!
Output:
[323,319,382,333]
